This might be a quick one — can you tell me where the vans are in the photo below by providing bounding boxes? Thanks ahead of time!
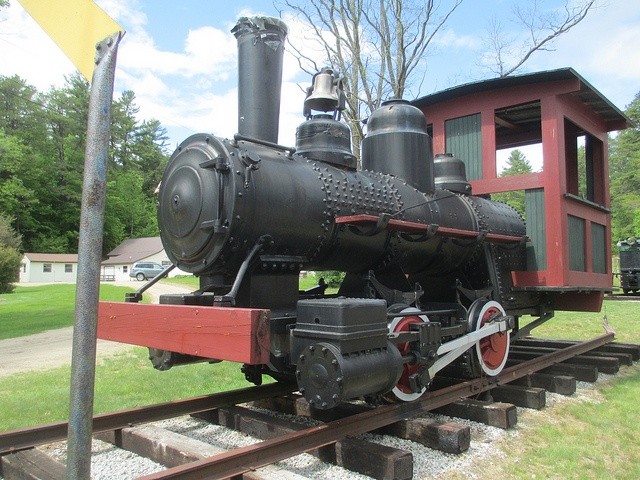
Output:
[129,262,168,281]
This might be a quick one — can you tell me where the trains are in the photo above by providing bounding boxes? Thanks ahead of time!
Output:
[124,14,640,410]
